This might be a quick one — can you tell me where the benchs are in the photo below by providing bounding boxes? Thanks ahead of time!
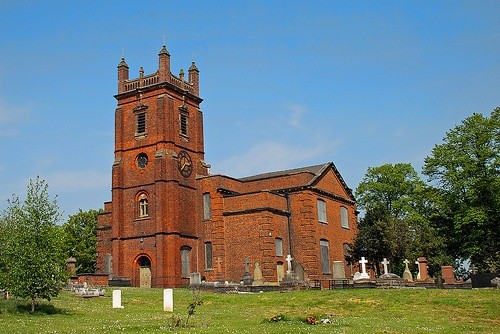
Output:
[329,280,349,289]
[308,279,321,290]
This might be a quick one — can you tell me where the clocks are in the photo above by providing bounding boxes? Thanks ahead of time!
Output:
[177,151,193,177]
